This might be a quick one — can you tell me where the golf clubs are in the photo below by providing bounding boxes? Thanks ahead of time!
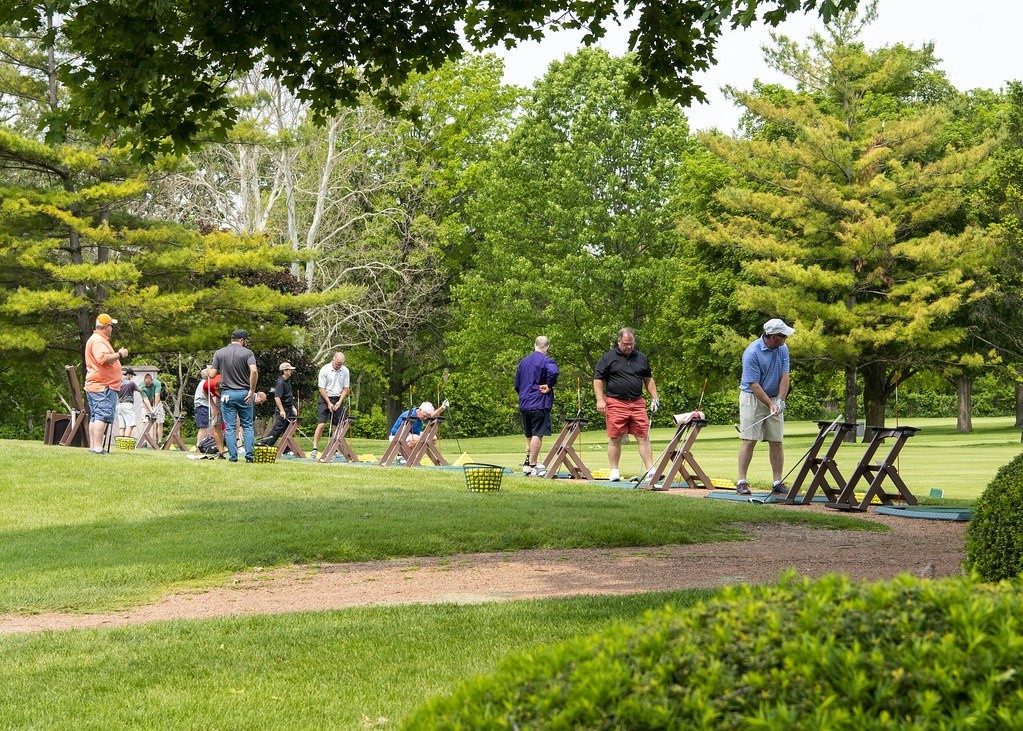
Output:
[381,405,413,467]
[328,411,333,463]
[734,411,777,433]
[633,417,697,489]
[208,435,274,460]
[160,411,197,452]
[446,406,462,455]
[537,409,581,477]
[320,406,348,463]
[748,414,844,505]
[276,409,313,444]
[628,409,655,483]
[536,449,546,469]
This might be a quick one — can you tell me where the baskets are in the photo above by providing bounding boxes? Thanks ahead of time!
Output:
[114,436,137,450]
[252,444,279,464]
[463,463,505,494]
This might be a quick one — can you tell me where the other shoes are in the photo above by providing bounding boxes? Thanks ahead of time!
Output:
[88,449,109,455]
[284,450,342,458]
[142,442,165,448]
[397,454,407,465]
[246,458,253,463]
[230,459,237,462]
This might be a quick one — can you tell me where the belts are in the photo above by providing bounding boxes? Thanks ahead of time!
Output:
[120,400,133,403]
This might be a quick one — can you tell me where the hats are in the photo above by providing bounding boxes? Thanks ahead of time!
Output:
[231,329,252,340]
[127,368,137,376]
[279,362,296,370]
[96,313,118,326]
[763,319,795,336]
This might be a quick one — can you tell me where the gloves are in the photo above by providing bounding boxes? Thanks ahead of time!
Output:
[776,400,785,413]
[292,405,298,415]
[651,401,658,414]
[441,399,450,410]
[221,395,229,402]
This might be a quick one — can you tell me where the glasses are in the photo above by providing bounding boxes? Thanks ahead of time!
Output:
[620,340,636,346]
[770,334,787,338]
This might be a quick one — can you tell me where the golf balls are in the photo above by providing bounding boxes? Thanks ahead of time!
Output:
[802,491,806,496]
[590,468,737,489]
[465,467,503,492]
[305,451,476,467]
[254,445,279,463]
[116,439,136,450]
[854,492,880,503]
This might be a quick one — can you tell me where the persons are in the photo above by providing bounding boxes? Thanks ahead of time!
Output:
[202,374,240,459]
[194,369,211,453]
[261,362,298,454]
[388,399,450,463]
[139,374,165,447]
[515,336,560,473]
[206,330,258,463]
[310,352,350,459]
[593,328,665,482]
[117,368,142,437]
[736,319,795,496]
[83,313,128,454]
[239,392,266,447]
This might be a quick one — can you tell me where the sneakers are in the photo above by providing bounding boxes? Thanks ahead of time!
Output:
[737,483,751,496]
[645,467,665,483]
[523,462,536,476]
[772,483,789,495]
[609,469,620,481]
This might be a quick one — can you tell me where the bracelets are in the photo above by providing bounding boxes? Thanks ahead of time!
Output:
[118,352,122,359]
